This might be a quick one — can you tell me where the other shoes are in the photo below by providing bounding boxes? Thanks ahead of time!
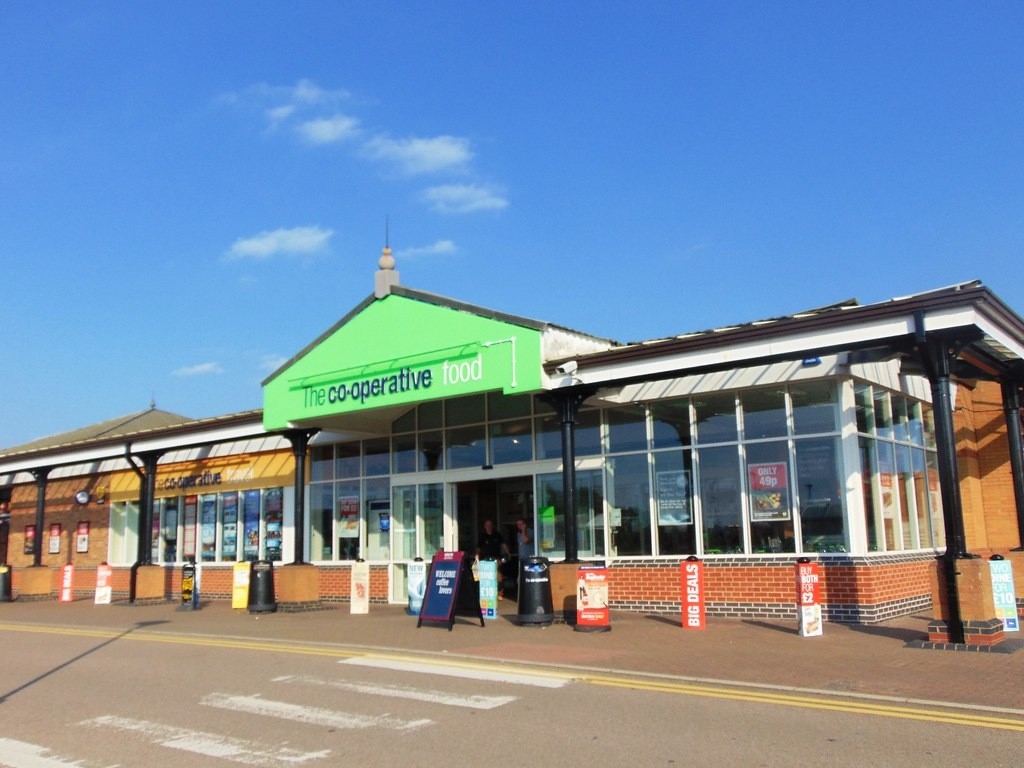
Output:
[498,593,503,600]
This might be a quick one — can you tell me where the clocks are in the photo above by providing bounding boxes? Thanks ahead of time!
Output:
[75,490,91,505]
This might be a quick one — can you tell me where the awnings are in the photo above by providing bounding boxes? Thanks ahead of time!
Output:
[107,449,310,504]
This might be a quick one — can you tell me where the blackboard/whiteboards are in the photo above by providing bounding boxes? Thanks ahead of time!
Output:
[423,560,460,619]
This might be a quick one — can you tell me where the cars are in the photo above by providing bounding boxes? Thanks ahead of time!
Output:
[802,504,844,547]
[708,523,756,550]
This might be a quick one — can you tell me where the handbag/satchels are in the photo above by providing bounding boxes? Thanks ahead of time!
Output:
[471,560,480,581]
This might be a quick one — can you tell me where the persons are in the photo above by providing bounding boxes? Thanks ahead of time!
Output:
[516,518,534,600]
[472,519,511,601]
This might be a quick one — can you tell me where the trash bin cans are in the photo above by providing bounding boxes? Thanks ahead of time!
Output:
[517,557,555,628]
[0,563,14,604]
[247,561,278,615]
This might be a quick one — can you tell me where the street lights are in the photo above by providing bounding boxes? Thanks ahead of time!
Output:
[807,484,813,499]
